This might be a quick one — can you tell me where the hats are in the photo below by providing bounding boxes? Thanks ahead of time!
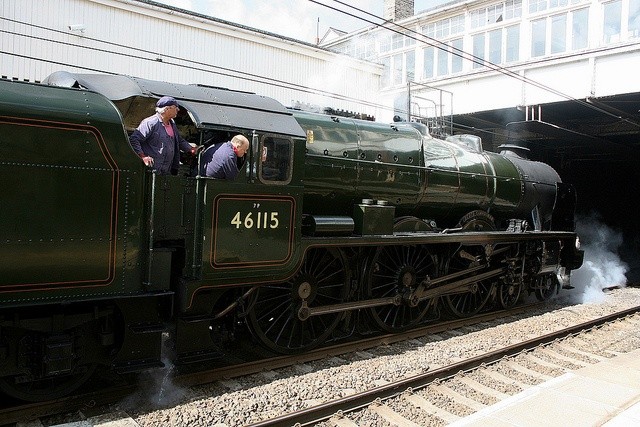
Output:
[155,96,178,107]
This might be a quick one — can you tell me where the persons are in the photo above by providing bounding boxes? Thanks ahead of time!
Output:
[191,134,250,179]
[129,96,199,176]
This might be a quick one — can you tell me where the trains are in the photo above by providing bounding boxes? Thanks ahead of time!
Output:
[0,70,585,402]
